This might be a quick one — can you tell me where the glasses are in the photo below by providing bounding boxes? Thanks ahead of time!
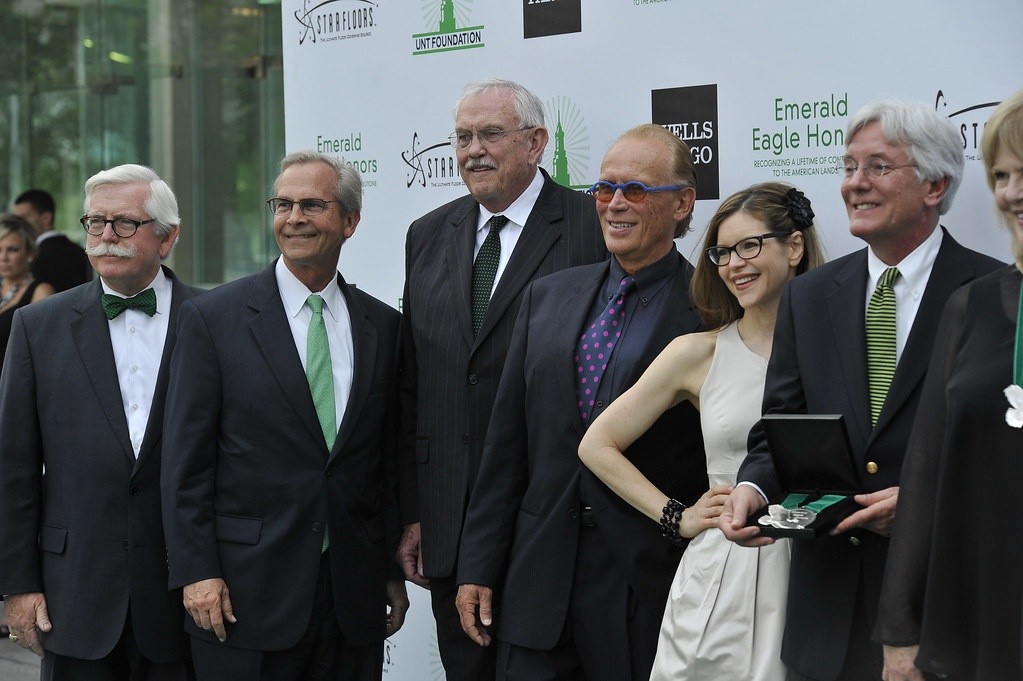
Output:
[704,230,794,267]
[448,124,537,152]
[80,212,155,239]
[590,179,684,204]
[267,196,340,217]
[835,155,919,182]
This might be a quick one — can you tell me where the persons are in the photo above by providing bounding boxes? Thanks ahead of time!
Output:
[868,98,1023,681]
[453,121,697,681]
[0,163,215,681]
[15,188,94,293]
[0,214,57,377]
[159,151,410,681]
[717,98,1010,681]
[576,181,826,681]
[396,77,610,681]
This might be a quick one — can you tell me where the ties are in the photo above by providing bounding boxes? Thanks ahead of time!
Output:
[866,266,902,428]
[471,215,510,341]
[306,293,337,454]
[576,274,638,430]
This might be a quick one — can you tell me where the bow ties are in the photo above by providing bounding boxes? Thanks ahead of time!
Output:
[101,286,156,320]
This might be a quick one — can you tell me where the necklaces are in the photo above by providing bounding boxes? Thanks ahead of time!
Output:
[0,271,32,310]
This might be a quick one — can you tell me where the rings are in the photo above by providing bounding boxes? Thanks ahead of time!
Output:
[9,634,17,641]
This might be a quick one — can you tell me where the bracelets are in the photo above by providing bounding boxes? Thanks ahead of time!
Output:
[660,499,686,541]
